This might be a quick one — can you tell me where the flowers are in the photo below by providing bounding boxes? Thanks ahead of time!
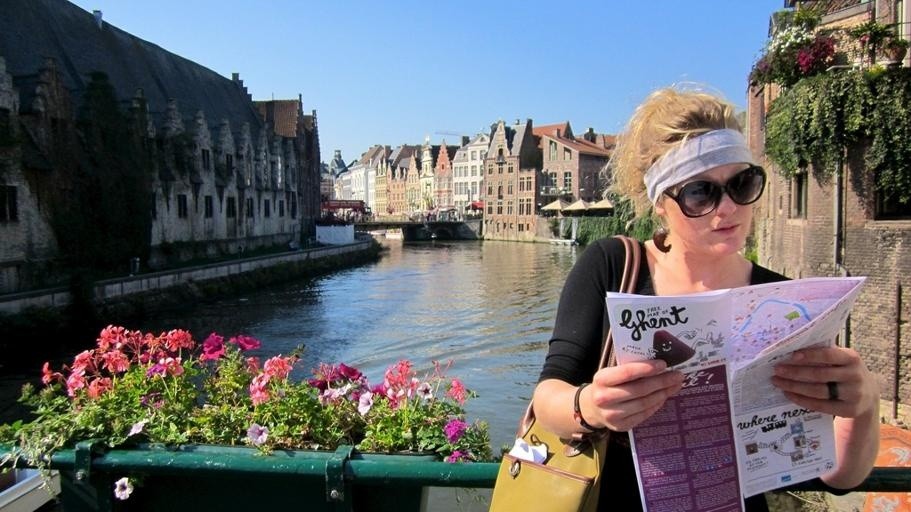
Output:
[0,324,511,501]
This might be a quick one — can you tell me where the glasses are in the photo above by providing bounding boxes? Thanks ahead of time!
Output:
[659,165,766,219]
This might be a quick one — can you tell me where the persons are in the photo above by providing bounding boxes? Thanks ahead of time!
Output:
[320,207,460,224]
[531,78,880,511]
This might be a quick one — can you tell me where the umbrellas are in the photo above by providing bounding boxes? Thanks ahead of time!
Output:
[538,198,617,213]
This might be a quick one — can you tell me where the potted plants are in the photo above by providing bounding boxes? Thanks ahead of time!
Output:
[884,40,911,63]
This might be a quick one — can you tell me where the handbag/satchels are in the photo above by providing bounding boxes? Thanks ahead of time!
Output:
[487,235,642,512]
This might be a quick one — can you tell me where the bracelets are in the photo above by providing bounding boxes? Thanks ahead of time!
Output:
[572,381,607,435]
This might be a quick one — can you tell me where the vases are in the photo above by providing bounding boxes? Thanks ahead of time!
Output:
[63,440,436,512]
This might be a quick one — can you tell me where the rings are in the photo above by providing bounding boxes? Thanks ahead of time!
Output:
[825,381,840,401]
